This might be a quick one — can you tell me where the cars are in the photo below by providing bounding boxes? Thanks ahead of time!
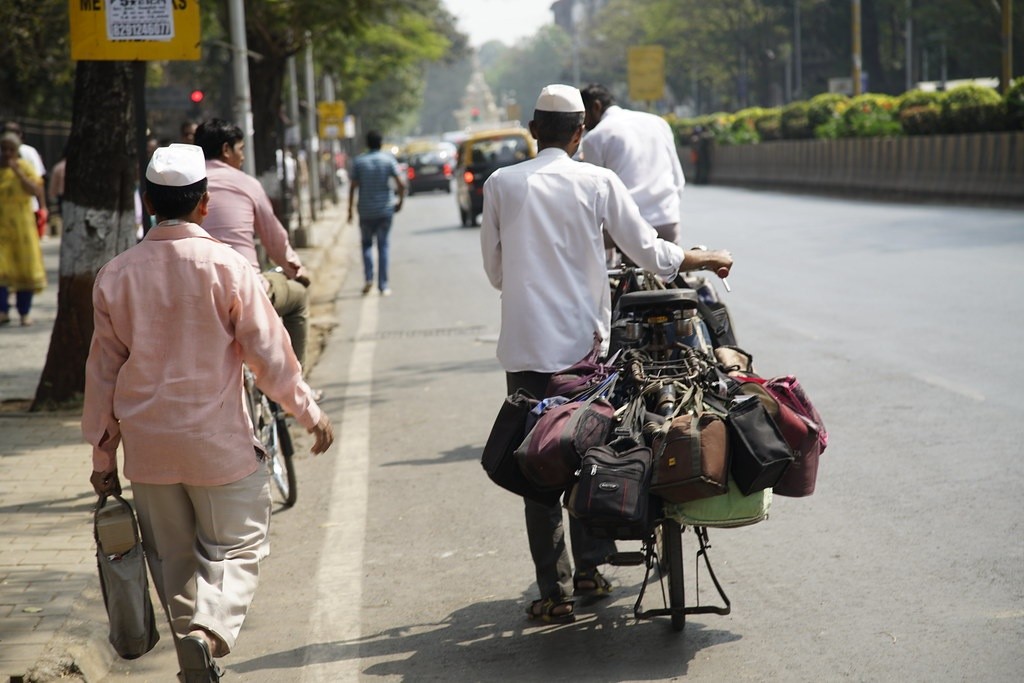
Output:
[407,152,451,195]
[444,153,459,174]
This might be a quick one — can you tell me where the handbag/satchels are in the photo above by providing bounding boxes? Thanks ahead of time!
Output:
[606,268,740,360]
[93,490,161,660]
[689,146,699,165]
[480,340,829,542]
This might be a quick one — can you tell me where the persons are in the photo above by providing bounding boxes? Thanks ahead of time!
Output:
[480,84,734,622]
[81,143,334,683]
[348,130,404,296]
[0,121,202,325]
[193,118,327,404]
[576,85,685,270]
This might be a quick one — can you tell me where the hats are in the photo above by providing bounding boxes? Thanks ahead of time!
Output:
[534,84,586,112]
[146,144,207,187]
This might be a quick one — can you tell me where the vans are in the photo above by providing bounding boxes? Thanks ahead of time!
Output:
[449,127,540,227]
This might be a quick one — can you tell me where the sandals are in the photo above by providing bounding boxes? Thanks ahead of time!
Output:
[523,594,576,625]
[572,563,614,597]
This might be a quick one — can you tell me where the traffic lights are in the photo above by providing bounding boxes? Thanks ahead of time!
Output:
[192,85,204,113]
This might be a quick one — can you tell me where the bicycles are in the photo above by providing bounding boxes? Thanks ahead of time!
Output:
[606,244,731,630]
[238,266,298,505]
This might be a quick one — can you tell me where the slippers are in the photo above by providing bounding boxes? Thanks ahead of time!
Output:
[177,636,226,683]
